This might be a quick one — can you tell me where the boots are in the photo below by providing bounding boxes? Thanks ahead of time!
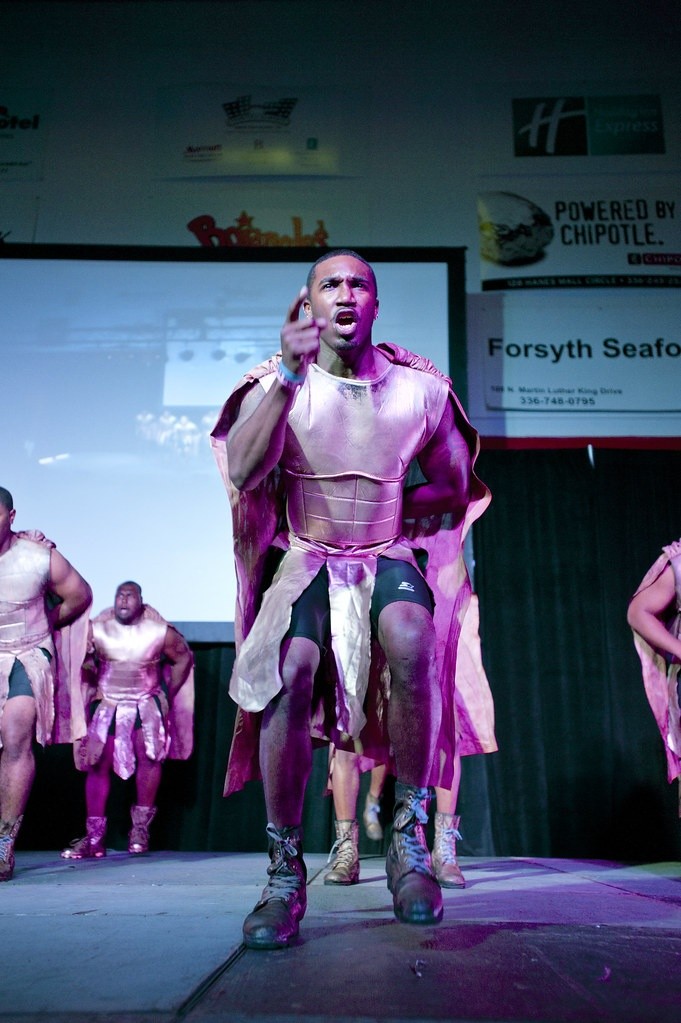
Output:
[60,816,107,859]
[324,818,360,886]
[363,792,383,841]
[128,805,156,855]
[431,812,466,889]
[0,815,23,881]
[385,781,444,926]
[242,824,307,949]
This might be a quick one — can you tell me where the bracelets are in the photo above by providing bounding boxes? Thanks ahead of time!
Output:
[277,360,306,390]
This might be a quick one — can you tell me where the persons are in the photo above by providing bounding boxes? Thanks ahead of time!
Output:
[136,408,219,459]
[211,250,491,950]
[0,488,93,881]
[60,581,195,859]
[309,591,499,889]
[626,541,680,788]
